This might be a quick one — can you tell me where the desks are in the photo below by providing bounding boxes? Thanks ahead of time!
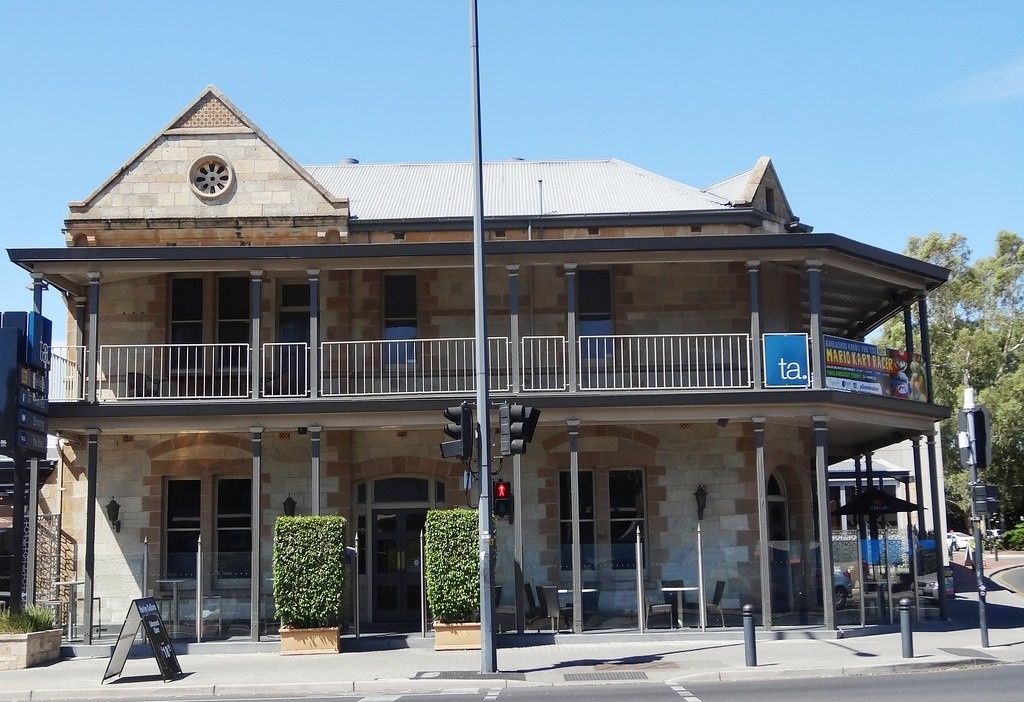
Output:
[661,587,700,628]
[557,589,599,593]
[55,577,189,640]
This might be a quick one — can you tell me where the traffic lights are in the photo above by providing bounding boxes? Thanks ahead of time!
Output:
[495,498,512,516]
[975,485,1000,516]
[494,481,511,497]
[499,403,527,457]
[439,402,473,462]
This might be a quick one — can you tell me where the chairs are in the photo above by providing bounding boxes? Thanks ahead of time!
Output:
[260,371,288,395]
[494,580,725,635]
[125,371,159,400]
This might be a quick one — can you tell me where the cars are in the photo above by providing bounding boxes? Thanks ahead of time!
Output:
[911,572,939,608]
[946,529,975,552]
[985,528,1002,543]
[833,565,853,611]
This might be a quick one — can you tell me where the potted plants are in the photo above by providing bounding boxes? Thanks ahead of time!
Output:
[0,601,64,670]
[424,508,498,651]
[272,515,348,655]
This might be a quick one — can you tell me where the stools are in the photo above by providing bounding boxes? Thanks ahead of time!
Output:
[200,596,222,636]
[74,597,102,638]
[143,597,172,639]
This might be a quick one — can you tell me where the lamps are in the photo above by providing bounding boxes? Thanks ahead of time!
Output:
[282,493,297,516]
[693,485,708,520]
[104,496,121,533]
